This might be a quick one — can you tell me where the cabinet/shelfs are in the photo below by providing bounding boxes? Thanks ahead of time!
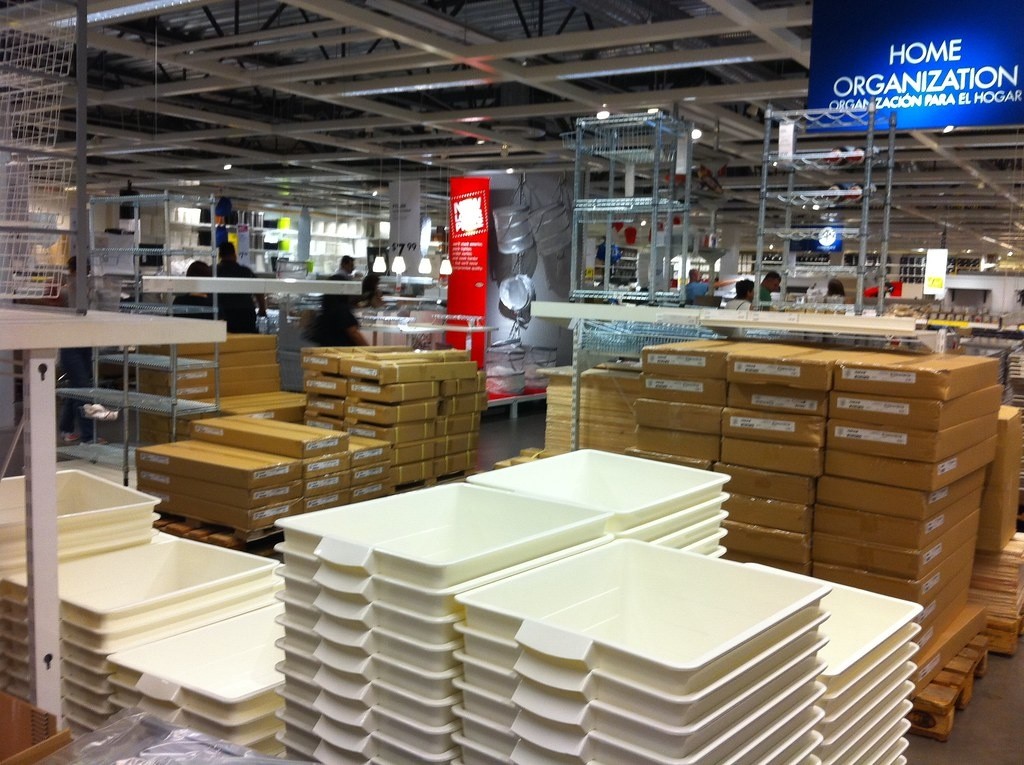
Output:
[54,187,218,486]
[747,94,899,313]
[570,110,694,304]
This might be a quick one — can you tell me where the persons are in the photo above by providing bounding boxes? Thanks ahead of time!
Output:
[686,269,737,305]
[725,279,755,310]
[214,241,266,335]
[169,260,215,319]
[313,275,371,348]
[54,255,108,449]
[761,272,781,310]
[356,276,382,308]
[337,255,356,280]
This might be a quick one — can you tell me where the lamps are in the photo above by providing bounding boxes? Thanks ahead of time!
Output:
[418,153,432,274]
[372,147,387,273]
[439,156,453,275]
[391,139,406,273]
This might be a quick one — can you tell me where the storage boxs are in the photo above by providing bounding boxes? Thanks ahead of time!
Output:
[0,444,933,765]
